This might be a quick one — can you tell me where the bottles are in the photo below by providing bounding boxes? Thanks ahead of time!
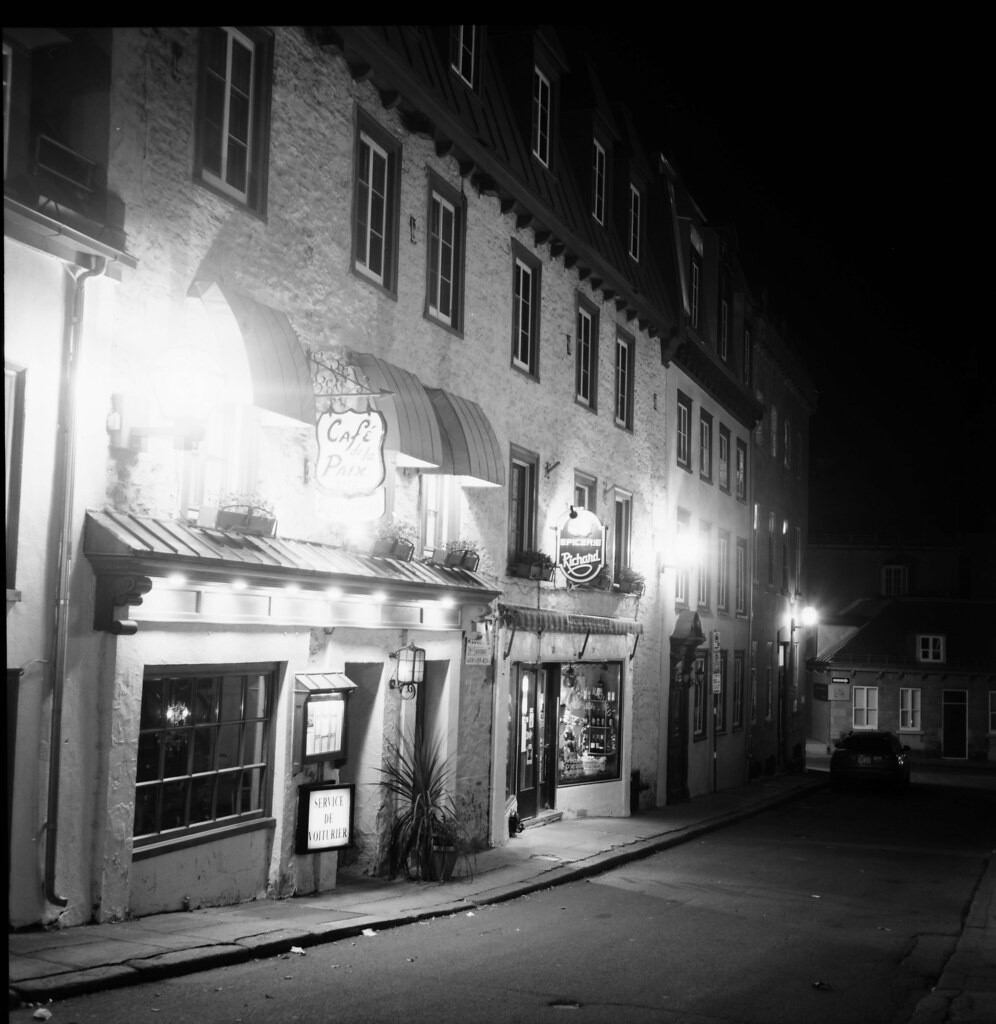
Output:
[583,713,612,753]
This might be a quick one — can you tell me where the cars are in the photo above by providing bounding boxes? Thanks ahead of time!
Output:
[830,729,912,794]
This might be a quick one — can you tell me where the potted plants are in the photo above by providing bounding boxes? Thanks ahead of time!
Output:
[429,539,483,571]
[618,566,648,596]
[517,549,555,580]
[215,489,278,544]
[372,716,477,887]
[366,518,419,563]
[586,560,612,591]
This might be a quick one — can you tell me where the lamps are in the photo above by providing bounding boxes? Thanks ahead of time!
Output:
[388,638,427,702]
[791,606,818,631]
